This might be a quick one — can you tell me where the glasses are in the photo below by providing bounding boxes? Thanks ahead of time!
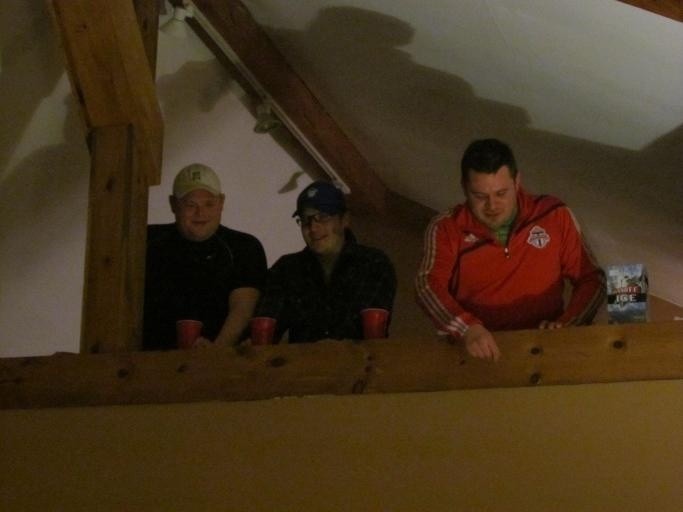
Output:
[295,210,336,227]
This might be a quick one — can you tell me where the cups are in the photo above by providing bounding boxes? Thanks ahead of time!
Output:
[358,307,389,339]
[174,319,203,350]
[247,317,276,345]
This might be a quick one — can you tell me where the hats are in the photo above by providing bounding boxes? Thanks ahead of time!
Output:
[171,164,222,200]
[292,181,347,219]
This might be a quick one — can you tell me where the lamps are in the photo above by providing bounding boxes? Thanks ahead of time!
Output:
[161,2,194,40]
[253,97,282,135]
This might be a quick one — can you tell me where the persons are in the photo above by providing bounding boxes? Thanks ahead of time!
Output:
[143,159,268,347]
[240,179,397,343]
[410,137,606,362]
